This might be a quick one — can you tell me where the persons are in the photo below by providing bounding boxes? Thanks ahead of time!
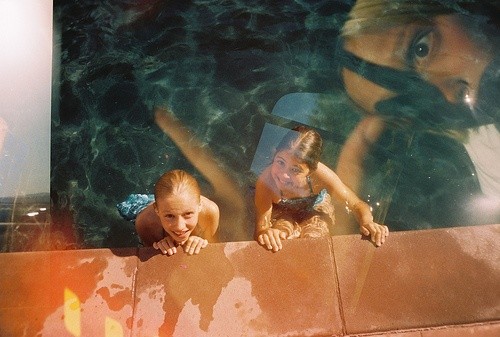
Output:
[135,169,220,256]
[254,125,389,254]
[154,0,500,235]
